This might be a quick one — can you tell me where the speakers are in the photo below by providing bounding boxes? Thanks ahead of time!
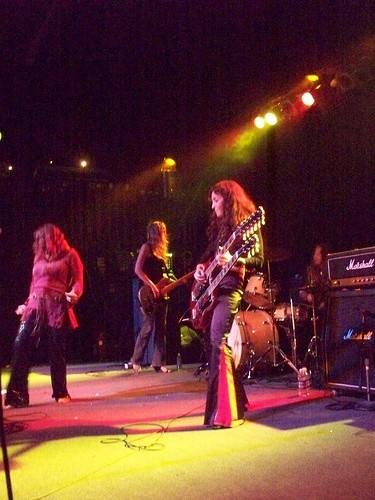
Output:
[324,289,375,393]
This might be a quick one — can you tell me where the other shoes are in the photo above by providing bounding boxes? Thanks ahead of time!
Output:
[58,397,70,403]
[131,357,141,371]
[153,365,172,373]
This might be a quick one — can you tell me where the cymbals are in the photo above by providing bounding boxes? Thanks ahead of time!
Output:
[297,283,321,291]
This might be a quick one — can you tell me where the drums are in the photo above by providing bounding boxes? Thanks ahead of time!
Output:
[269,301,311,323]
[227,310,279,373]
[307,336,322,360]
[243,271,281,309]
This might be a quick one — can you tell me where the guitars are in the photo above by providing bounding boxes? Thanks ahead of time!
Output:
[138,269,196,314]
[189,207,265,328]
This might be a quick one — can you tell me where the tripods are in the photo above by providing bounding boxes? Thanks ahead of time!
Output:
[242,260,321,386]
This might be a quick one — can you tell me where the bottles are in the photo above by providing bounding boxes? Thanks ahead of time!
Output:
[303,368,310,395]
[298,369,307,397]
[177,353,182,371]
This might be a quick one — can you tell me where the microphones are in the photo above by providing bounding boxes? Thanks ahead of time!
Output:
[360,309,367,325]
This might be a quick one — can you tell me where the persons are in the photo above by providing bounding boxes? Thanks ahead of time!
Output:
[194,181,264,429]
[2,222,83,410]
[300,242,336,311]
[131,221,176,373]
